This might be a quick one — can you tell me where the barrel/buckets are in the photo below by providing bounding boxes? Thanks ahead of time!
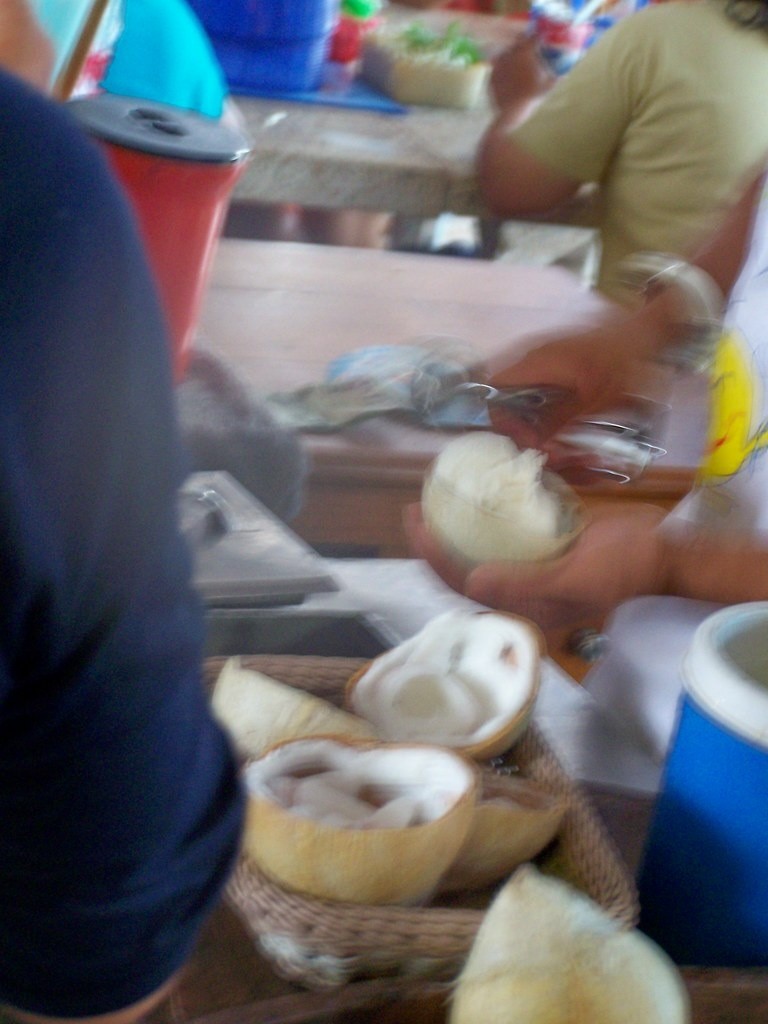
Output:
[634,601,767,977]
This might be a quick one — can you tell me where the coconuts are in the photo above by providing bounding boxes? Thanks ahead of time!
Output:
[211,609,570,905]
[423,429,593,564]
[442,859,693,1023]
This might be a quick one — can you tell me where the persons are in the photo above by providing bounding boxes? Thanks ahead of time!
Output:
[0,0,232,119]
[1,64,251,1024]
[401,165,768,628]
[476,0,768,306]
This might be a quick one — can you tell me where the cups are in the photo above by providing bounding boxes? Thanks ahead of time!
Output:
[74,97,254,367]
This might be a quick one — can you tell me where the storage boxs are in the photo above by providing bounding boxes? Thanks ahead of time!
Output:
[361,34,490,107]
[187,0,341,89]
[330,17,383,62]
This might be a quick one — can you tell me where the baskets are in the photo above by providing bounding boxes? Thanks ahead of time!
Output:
[179,650,640,986]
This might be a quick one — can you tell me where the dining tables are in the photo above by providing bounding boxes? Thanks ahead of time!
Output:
[142,70,690,565]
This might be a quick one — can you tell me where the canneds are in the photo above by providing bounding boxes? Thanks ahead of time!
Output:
[538,11,588,75]
